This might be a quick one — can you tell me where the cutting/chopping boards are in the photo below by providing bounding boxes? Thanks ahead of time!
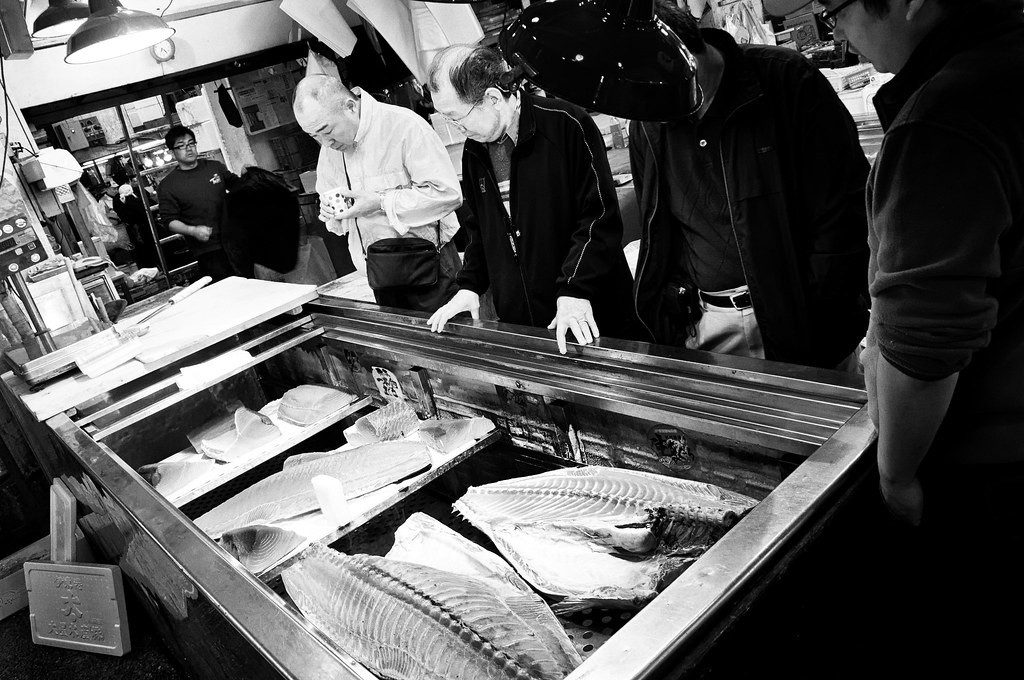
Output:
[112,276,318,363]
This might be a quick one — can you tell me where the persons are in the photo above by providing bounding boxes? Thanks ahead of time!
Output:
[100,173,170,267]
[427,44,639,356]
[292,73,464,313]
[819,1,1023,680]
[156,125,255,282]
[499,0,874,377]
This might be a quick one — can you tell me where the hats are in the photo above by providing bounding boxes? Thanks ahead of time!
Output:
[106,181,119,188]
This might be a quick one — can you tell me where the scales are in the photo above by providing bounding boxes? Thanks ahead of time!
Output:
[0,213,120,387]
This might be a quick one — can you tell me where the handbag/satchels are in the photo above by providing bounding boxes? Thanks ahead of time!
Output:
[365,238,440,290]
[76,181,118,243]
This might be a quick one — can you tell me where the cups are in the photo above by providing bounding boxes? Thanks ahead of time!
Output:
[319,187,353,220]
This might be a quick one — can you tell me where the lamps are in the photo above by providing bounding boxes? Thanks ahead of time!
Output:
[65,0,176,65]
[31,0,89,38]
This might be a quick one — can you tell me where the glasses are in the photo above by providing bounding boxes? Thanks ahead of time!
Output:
[819,0,856,29]
[441,86,495,133]
[172,141,197,150]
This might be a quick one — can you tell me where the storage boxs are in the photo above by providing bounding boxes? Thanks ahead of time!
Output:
[826,64,876,93]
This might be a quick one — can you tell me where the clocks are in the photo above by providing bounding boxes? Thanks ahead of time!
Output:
[150,37,175,64]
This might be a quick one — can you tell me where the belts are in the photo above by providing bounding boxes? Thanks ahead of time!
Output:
[699,291,752,311]
